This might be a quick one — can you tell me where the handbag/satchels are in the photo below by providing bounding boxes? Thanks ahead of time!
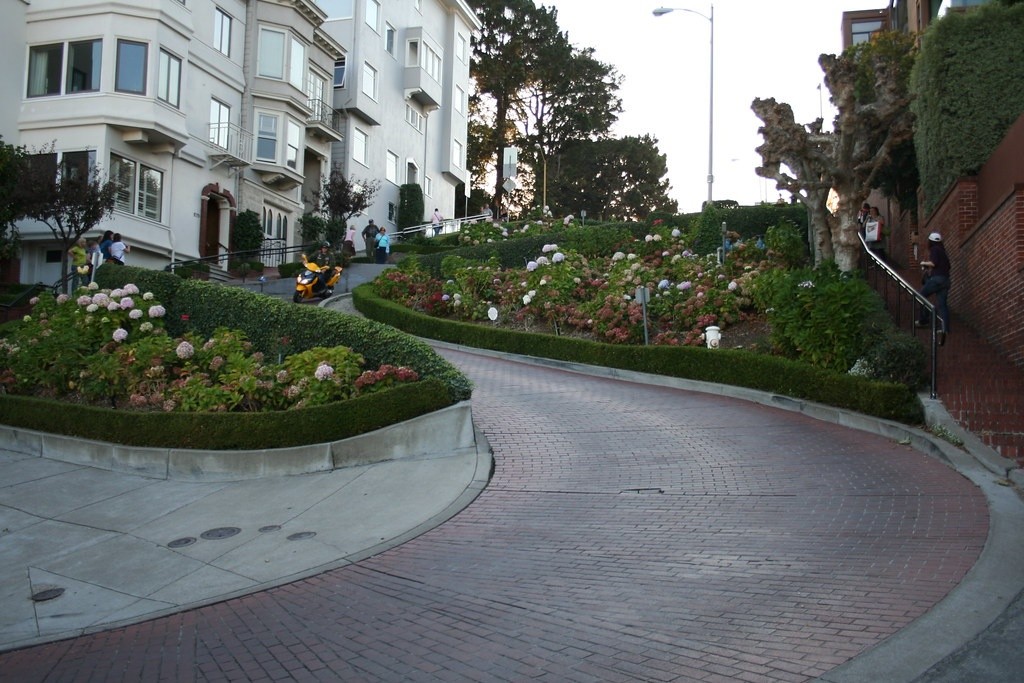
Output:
[351,244,355,253]
[105,258,116,264]
[866,217,881,241]
[439,220,443,228]
[374,241,379,248]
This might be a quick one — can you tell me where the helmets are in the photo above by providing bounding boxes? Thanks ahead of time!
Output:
[322,241,330,247]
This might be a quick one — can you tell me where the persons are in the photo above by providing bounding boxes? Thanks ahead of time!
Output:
[857,203,889,268]
[432,208,444,237]
[68,230,131,293]
[915,232,952,335]
[479,203,508,222]
[343,219,391,263]
[303,241,336,293]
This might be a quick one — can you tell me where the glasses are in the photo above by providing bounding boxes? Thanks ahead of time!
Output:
[322,246,327,249]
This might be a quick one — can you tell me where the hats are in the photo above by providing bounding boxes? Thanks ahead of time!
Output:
[369,219,373,223]
[928,233,941,242]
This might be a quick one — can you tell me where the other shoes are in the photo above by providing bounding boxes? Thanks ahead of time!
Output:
[937,329,949,335]
[319,287,327,294]
[915,321,928,328]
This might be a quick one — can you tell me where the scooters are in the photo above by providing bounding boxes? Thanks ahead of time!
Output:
[293,253,343,305]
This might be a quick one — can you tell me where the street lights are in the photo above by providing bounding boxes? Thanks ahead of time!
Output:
[651,3,714,206]
